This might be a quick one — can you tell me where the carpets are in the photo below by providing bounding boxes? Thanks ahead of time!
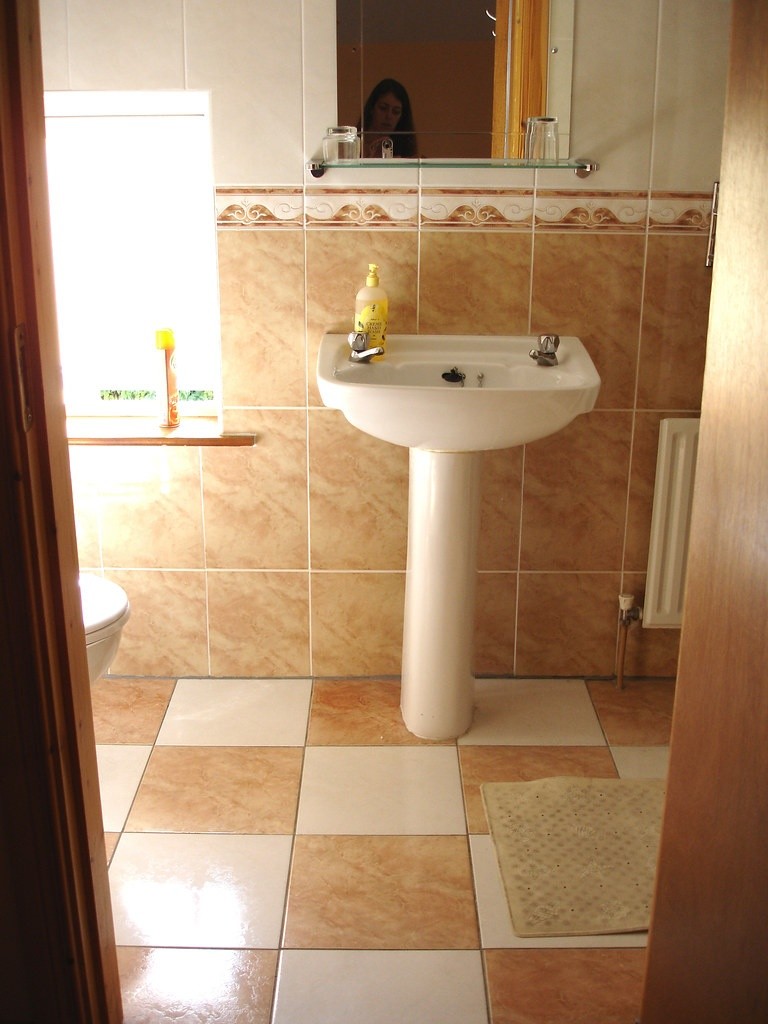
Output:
[479,778,666,937]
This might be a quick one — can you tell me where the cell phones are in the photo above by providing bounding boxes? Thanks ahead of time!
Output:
[382,139,393,158]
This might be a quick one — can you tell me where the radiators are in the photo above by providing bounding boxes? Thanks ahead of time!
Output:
[616,417,699,694]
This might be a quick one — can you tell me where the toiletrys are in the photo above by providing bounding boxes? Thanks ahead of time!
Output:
[354,263,389,362]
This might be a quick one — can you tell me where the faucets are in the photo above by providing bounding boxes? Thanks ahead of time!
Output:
[347,331,385,364]
[529,334,560,367]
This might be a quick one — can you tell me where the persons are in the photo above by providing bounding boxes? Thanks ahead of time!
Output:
[356,79,416,158]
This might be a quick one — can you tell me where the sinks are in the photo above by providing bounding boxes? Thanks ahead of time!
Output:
[316,333,602,453]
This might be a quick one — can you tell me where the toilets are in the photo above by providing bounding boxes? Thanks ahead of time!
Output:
[78,573,131,687]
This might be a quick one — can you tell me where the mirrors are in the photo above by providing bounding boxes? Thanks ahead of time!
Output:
[336,0,575,160]
